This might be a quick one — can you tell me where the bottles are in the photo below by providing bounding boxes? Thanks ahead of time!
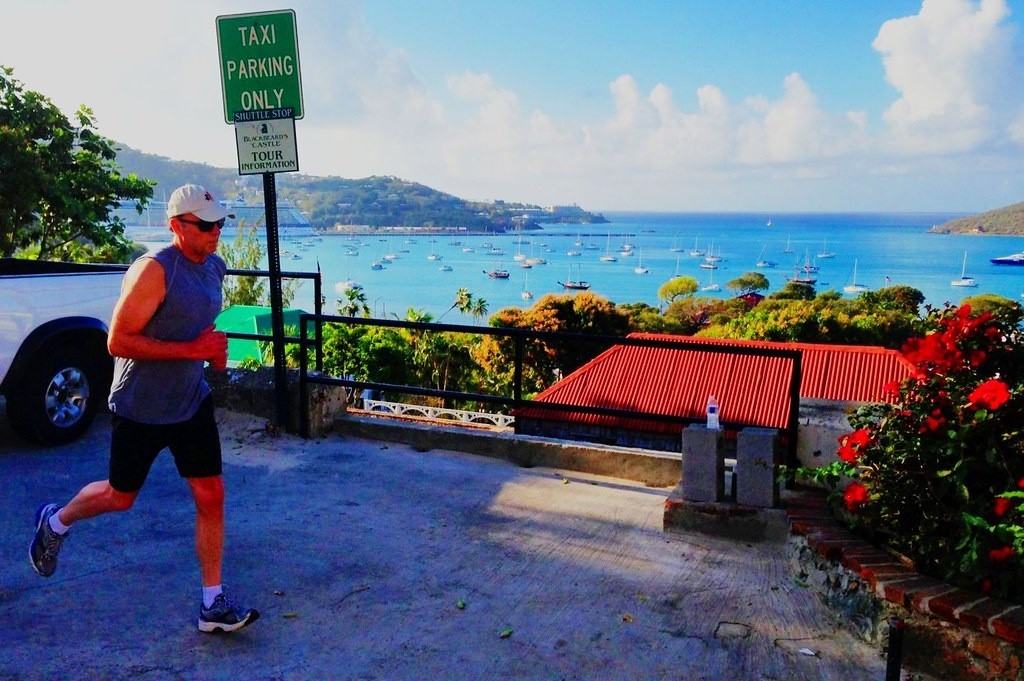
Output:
[706,396,719,428]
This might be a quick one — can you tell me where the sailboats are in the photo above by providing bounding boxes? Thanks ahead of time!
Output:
[843,258,872,292]
[670,235,724,293]
[567,225,635,262]
[429,220,549,277]
[521,274,534,299]
[784,237,819,285]
[557,262,591,289]
[950,251,980,287]
[817,241,838,259]
[635,246,651,273]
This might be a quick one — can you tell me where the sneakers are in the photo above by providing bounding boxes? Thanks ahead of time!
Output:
[29,503,69,578]
[199,593,260,634]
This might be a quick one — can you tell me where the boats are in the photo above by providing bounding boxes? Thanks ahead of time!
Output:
[756,261,778,268]
[279,231,323,260]
[342,218,419,271]
[990,252,1024,266]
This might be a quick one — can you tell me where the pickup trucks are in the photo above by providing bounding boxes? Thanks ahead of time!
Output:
[0,256,133,444]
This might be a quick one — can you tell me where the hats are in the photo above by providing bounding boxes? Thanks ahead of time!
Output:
[168,183,235,222]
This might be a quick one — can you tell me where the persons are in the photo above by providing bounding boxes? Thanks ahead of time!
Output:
[28,184,260,634]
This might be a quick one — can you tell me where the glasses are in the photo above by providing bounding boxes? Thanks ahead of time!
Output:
[180,218,223,232]
